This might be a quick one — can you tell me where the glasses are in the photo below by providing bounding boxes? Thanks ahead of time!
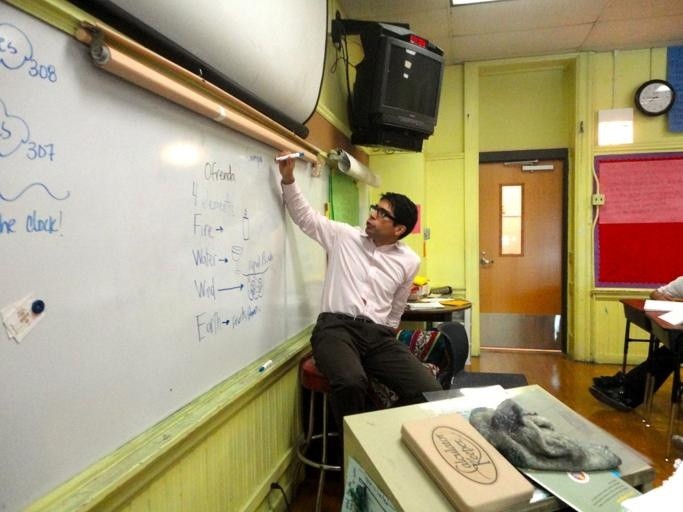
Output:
[370,205,395,221]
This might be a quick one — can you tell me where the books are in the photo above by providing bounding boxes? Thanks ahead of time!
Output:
[644,299,683,328]
[405,302,445,311]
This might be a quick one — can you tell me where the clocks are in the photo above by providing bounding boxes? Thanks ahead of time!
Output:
[635,79,676,117]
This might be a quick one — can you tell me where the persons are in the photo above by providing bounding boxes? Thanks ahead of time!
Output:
[590,276,683,412]
[275,150,442,413]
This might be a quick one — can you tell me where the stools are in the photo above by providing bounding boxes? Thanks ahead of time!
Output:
[295,348,344,511]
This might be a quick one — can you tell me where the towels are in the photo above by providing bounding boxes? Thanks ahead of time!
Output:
[468,399,622,472]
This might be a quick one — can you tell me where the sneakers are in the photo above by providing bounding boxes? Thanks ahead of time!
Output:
[589,371,636,412]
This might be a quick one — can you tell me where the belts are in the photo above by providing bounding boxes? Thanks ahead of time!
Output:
[318,312,373,324]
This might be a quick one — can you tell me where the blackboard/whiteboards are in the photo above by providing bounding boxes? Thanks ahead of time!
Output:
[0,0,334,512]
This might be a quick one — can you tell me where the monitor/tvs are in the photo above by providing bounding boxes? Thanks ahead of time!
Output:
[369,36,445,125]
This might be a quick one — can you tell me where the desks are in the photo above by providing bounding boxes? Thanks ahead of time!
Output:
[396,293,471,332]
[341,383,656,512]
[617,296,683,461]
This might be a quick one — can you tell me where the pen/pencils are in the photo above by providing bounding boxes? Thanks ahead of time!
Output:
[259,360,273,373]
[275,152,304,162]
